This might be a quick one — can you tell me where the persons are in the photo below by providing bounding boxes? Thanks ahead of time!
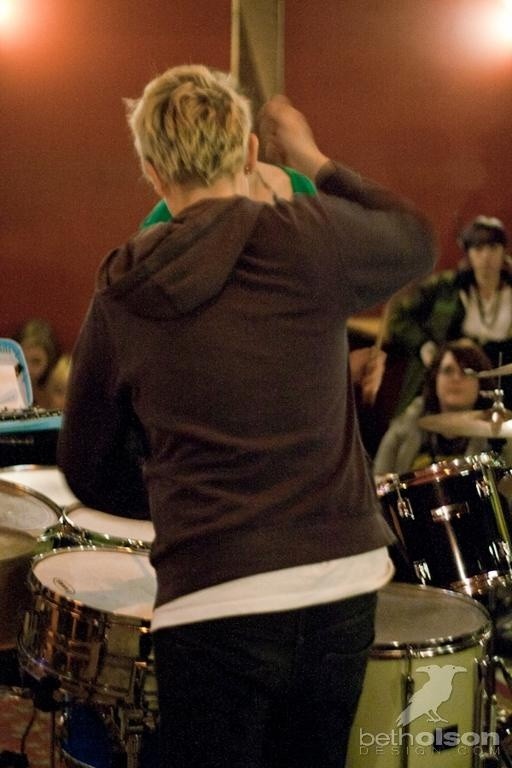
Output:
[0,212,511,492]
[55,61,445,766]
[139,155,321,234]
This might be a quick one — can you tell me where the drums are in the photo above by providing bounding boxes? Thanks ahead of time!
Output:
[344,583,497,762]
[16,544,160,711]
[374,450,512,596]
[0,478,64,652]
[58,503,156,550]
[0,461,82,508]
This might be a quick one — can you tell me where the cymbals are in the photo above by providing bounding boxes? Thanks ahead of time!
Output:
[417,408,512,439]
[479,359,512,379]
